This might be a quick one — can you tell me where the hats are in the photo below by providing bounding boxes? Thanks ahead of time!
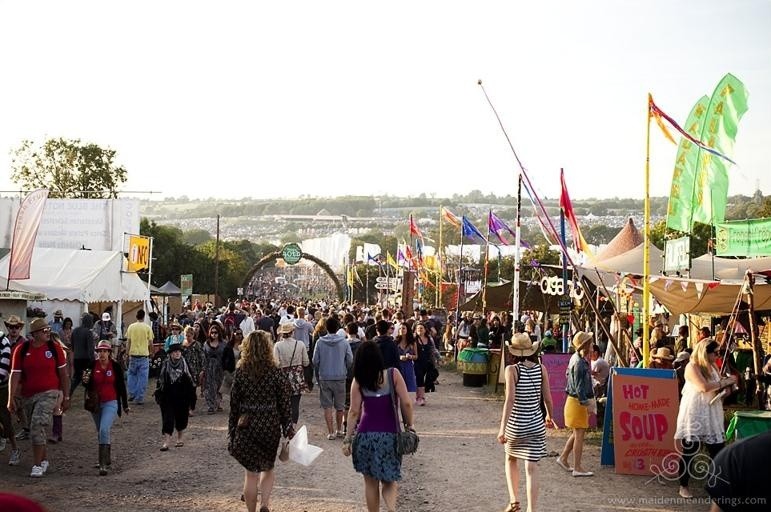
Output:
[52,310,63,318]
[2,314,25,331]
[100,312,112,322]
[571,330,595,352]
[28,317,52,334]
[168,321,183,329]
[506,332,540,359]
[652,347,675,361]
[545,330,551,337]
[459,312,481,320]
[277,323,295,334]
[164,343,185,354]
[674,351,690,364]
[94,340,113,353]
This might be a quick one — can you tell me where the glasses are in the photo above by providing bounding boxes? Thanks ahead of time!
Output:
[6,324,21,331]
[36,327,51,332]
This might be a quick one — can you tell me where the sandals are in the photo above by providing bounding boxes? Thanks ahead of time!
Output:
[159,446,169,451]
[174,441,185,448]
[504,501,521,512]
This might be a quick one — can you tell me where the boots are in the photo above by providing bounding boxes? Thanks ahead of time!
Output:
[91,443,111,476]
[46,414,64,444]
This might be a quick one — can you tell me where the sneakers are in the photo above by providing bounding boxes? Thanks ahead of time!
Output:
[0,437,7,451]
[327,429,343,440]
[207,405,224,415]
[40,459,50,473]
[14,427,30,441]
[30,464,43,478]
[6,446,25,467]
[126,395,146,406]
[414,396,426,407]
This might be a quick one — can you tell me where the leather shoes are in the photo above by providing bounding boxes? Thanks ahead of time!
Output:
[677,487,695,501]
[570,469,595,478]
[556,457,574,472]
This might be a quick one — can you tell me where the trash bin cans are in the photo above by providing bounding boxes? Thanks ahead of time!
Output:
[458,348,489,387]
[726,411,771,441]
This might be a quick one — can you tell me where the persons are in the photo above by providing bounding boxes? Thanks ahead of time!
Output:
[702,432,770,511]
[674,338,729,499]
[557,329,597,479]
[79,337,131,478]
[340,338,418,512]
[498,330,555,512]
[7,317,72,479]
[225,329,297,511]
[0,283,771,468]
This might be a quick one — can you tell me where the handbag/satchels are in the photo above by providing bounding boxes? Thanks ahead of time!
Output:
[217,374,235,396]
[395,432,419,455]
[83,391,102,414]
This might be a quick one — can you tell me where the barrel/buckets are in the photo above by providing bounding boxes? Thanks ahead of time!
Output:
[462,348,489,388]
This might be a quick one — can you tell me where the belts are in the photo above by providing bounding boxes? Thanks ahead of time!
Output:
[130,354,149,359]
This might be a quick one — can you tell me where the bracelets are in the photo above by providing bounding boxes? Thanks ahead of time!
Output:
[344,435,353,445]
[65,396,70,401]
[409,422,416,430]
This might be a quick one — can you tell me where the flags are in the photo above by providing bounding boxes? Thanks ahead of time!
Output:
[664,94,713,238]
[347,168,595,283]
[648,91,741,167]
[689,72,750,229]
